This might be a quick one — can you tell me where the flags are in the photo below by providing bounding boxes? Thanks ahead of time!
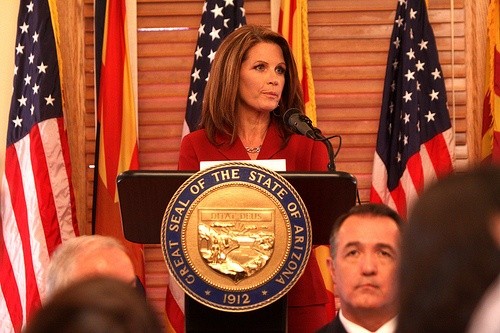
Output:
[368,0,455,222]
[270,0,318,128]
[93,0,145,308]
[0,0,81,333]
[165,1,246,333]
[480,0,500,168]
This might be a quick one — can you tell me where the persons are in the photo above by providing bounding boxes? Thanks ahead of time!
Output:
[21,165,500,333]
[177,24,330,173]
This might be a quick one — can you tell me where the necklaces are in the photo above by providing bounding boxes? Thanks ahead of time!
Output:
[245,145,263,154]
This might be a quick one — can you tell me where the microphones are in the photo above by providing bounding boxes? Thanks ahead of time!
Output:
[282,108,317,140]
